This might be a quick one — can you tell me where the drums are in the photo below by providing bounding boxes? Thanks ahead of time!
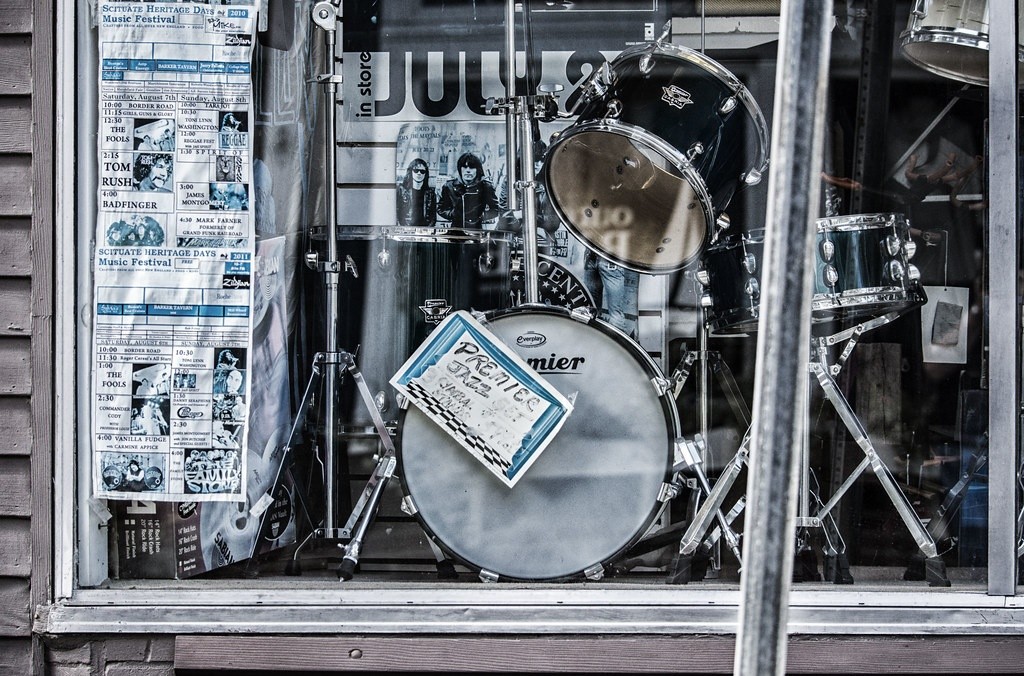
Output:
[394,300,684,587]
[896,0,1023,89]
[703,213,930,335]
[543,38,767,278]
[364,225,517,322]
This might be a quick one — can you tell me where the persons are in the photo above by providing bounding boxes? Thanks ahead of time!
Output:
[134,153,171,193]
[494,140,562,259]
[138,128,174,151]
[397,158,438,227]
[438,151,500,230]
[582,247,640,337]
[120,348,246,496]
[106,224,166,246]
[220,112,241,132]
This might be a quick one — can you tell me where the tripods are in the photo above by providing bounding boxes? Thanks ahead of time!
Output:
[240,11,456,584]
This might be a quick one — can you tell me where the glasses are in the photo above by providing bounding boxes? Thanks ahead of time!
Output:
[533,154,547,163]
[411,168,426,174]
